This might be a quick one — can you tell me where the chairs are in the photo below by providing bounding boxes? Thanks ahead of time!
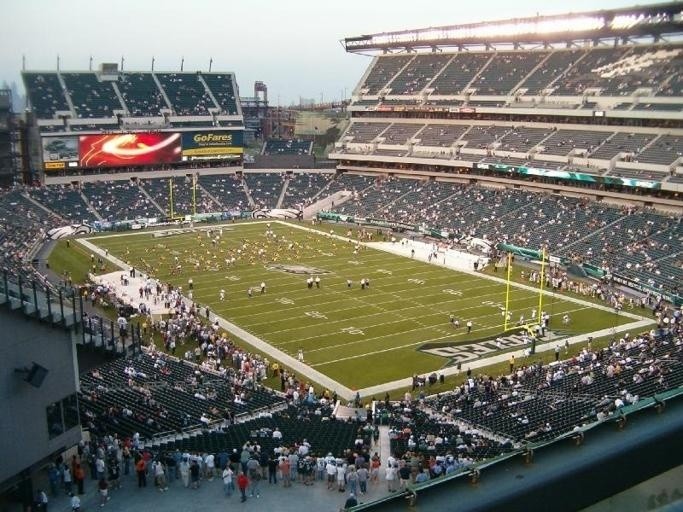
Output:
[387,313,682,470]
[1,264,385,471]
[0,52,335,263]
[336,51,683,307]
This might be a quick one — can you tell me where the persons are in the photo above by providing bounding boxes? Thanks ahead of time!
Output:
[0,53,682,511]
